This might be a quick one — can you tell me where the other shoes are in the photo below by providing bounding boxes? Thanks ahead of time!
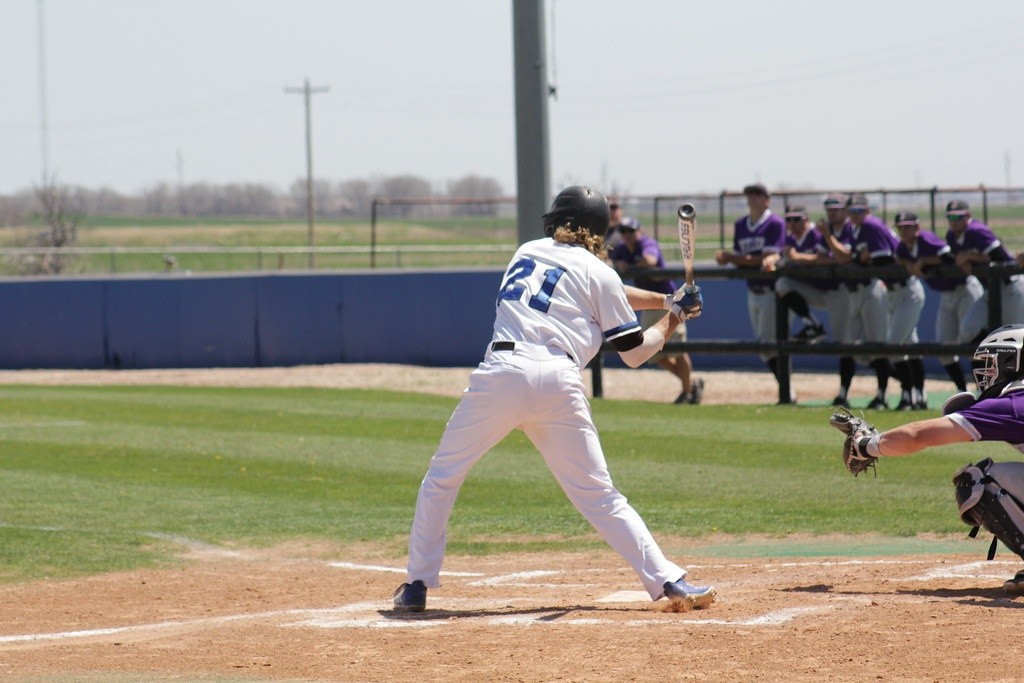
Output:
[830,395,849,407]
[675,392,692,404]
[1003,573,1024,593]
[690,378,705,404]
[868,397,887,410]
[914,401,927,410]
[791,323,826,343]
[895,400,914,410]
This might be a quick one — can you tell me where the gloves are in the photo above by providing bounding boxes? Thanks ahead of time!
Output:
[663,280,703,324]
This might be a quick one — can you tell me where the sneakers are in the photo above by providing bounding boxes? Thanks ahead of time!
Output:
[663,579,717,609]
[393,580,427,611]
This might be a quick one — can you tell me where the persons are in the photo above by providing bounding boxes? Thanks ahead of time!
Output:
[392,185,717,613]
[600,204,641,367]
[828,324,1024,591]
[716,183,786,382]
[606,217,705,404]
[772,192,1024,411]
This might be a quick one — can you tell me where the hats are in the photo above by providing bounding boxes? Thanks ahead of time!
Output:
[784,205,808,220]
[895,211,919,226]
[823,194,845,210]
[845,196,868,210]
[946,200,969,216]
[743,182,770,199]
[616,217,639,229]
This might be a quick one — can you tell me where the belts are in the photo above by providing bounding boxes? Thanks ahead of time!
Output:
[491,342,573,360]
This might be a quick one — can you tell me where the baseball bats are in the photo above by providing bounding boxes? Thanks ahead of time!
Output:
[678,203,696,286]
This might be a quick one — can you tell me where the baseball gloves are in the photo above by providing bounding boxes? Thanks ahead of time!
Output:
[829,413,880,475]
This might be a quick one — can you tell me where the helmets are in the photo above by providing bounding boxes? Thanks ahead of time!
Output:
[971,323,1024,398]
[543,185,611,249]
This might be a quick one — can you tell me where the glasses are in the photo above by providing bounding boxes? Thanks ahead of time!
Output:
[785,216,801,222]
